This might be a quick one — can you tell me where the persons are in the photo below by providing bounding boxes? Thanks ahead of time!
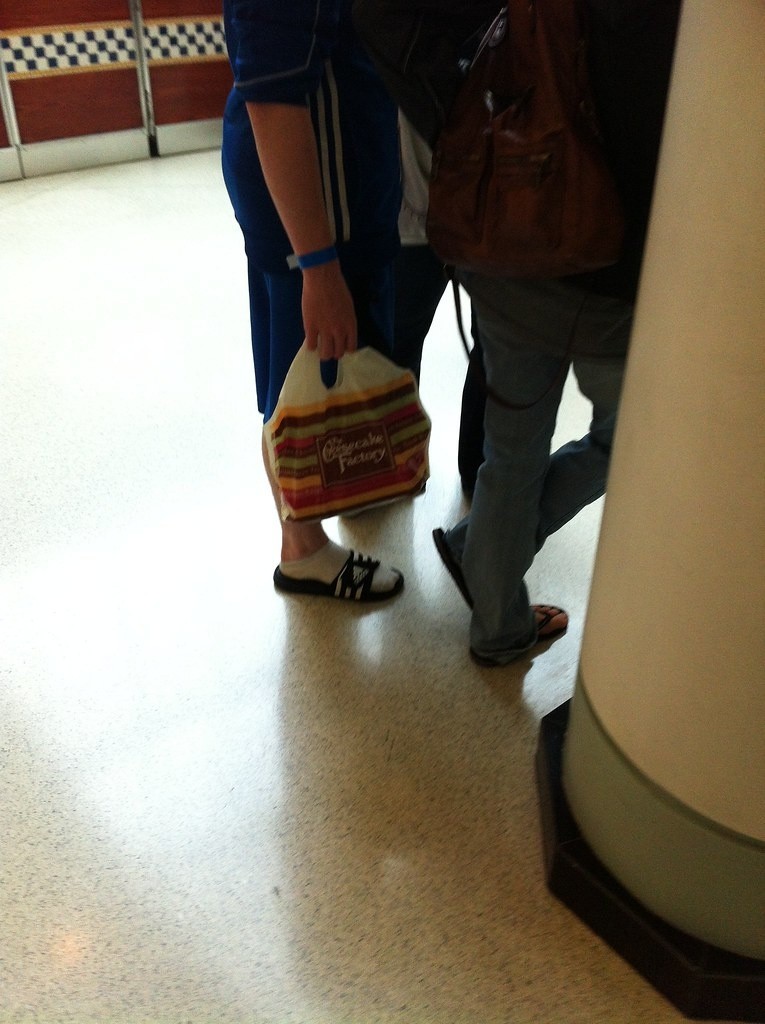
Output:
[220,1,451,609]
[429,0,683,671]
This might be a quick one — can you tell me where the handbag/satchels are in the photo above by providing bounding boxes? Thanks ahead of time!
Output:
[263,333,431,524]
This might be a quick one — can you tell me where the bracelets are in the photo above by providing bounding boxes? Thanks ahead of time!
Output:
[298,246,337,271]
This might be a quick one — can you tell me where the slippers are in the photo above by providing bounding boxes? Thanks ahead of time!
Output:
[274,550,404,602]
[433,528,474,609]
[469,605,567,667]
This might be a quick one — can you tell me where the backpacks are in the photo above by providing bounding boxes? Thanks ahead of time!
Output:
[350,0,509,146]
